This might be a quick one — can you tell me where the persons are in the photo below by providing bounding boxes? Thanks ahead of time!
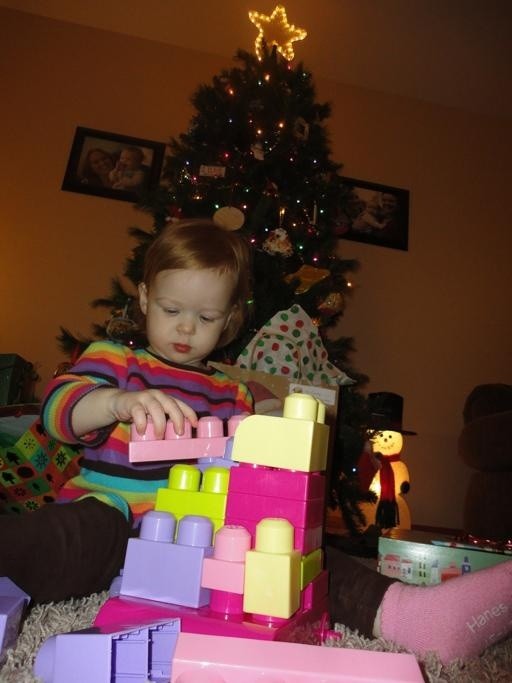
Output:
[85,147,148,192]
[0,217,512,674]
[353,192,397,237]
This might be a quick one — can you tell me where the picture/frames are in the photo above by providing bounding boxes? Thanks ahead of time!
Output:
[327,172,412,252]
[62,126,168,206]
